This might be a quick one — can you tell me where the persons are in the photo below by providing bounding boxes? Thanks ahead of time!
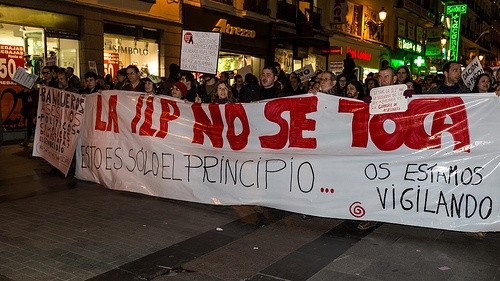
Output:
[427,61,471,94]
[248,65,291,103]
[16,53,500,238]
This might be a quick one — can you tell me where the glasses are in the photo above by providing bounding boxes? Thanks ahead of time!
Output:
[170,87,180,91]
[319,78,332,82]
[42,71,51,75]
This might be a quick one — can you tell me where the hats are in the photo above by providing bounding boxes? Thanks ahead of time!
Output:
[175,81,189,97]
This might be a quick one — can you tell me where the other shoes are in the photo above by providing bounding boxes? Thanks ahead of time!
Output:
[464,232,486,241]
[68,176,77,185]
[358,221,377,229]
[300,214,313,220]
